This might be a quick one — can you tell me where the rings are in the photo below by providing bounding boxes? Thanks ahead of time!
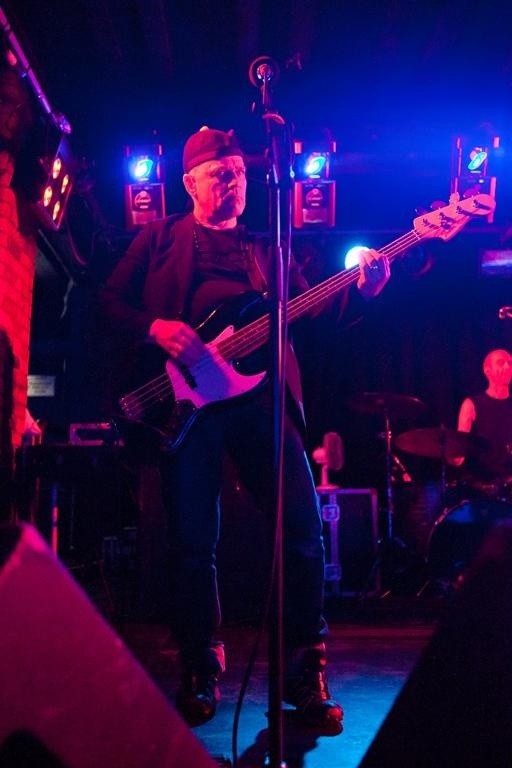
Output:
[370,266,379,277]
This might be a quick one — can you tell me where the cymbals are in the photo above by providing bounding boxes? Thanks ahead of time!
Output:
[345,392,424,421]
[393,426,490,459]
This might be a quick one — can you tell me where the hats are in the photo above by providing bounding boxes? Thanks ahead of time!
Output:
[182,126,245,173]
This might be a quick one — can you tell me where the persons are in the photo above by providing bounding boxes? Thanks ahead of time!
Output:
[93,128,395,730]
[439,347,512,496]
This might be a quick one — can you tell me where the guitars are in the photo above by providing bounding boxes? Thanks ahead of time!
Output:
[108,187,494,461]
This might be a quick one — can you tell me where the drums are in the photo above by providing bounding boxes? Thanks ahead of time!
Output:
[404,484,491,574]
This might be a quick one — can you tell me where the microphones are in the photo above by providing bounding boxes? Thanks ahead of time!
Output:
[24,417,49,455]
[247,54,294,185]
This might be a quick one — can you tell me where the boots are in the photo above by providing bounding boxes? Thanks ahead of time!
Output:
[175,642,227,724]
[290,644,344,725]
